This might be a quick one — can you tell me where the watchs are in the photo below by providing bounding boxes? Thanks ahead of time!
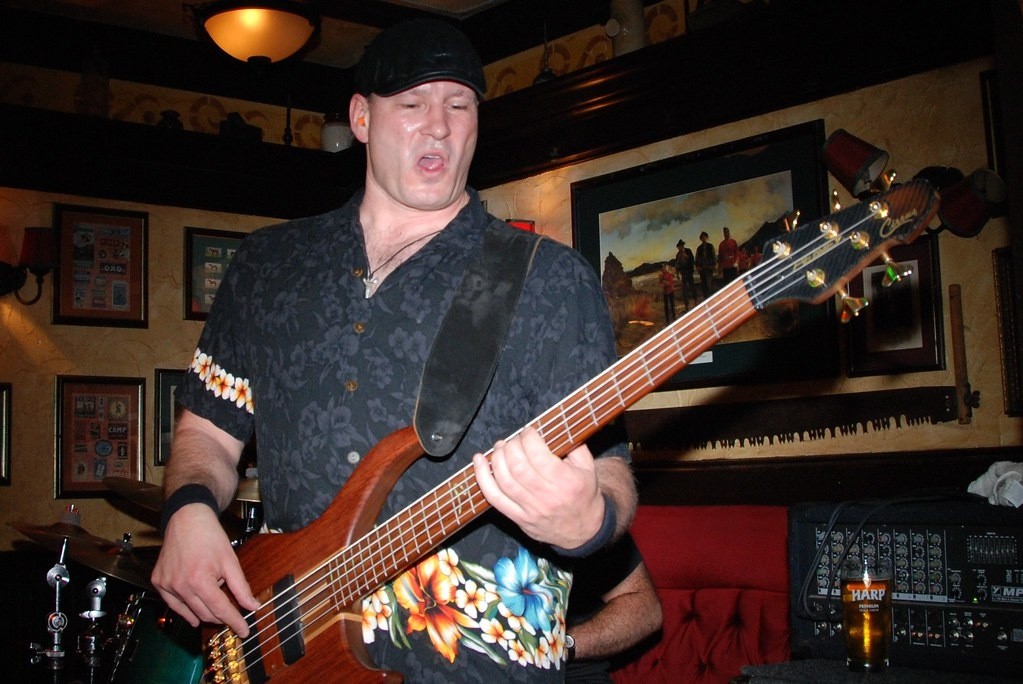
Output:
[560,632,577,664]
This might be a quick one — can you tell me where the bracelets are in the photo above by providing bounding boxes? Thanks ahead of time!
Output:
[549,492,618,557]
[159,483,221,537]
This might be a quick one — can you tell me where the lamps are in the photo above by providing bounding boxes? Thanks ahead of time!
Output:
[818,128,904,205]
[0,227,53,306]
[203,0,316,69]
[926,163,1004,241]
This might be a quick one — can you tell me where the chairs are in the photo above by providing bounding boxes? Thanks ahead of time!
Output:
[609,506,792,684]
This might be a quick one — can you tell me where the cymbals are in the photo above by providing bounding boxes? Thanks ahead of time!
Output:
[6,520,115,551]
[70,537,157,594]
[234,476,263,505]
[102,474,249,543]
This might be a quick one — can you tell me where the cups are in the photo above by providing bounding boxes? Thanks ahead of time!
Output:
[839,558,893,673]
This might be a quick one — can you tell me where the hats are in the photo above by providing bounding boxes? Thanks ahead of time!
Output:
[356,20,486,102]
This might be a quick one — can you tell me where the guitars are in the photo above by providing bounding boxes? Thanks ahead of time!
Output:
[194,164,950,683]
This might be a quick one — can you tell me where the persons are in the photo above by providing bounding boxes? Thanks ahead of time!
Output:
[674,238,695,313]
[151,18,663,684]
[718,226,739,285]
[694,226,716,297]
[656,260,677,332]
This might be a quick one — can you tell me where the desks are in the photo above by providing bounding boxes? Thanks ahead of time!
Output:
[732,658,1012,684]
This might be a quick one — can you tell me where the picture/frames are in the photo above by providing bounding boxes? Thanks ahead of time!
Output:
[49,201,150,330]
[154,369,195,466]
[0,382,13,486]
[571,118,831,393]
[53,374,146,498]
[182,226,249,322]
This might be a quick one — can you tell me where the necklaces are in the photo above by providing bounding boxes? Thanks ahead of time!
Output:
[358,219,448,299]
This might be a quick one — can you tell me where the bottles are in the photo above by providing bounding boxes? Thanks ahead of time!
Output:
[611,0,646,58]
